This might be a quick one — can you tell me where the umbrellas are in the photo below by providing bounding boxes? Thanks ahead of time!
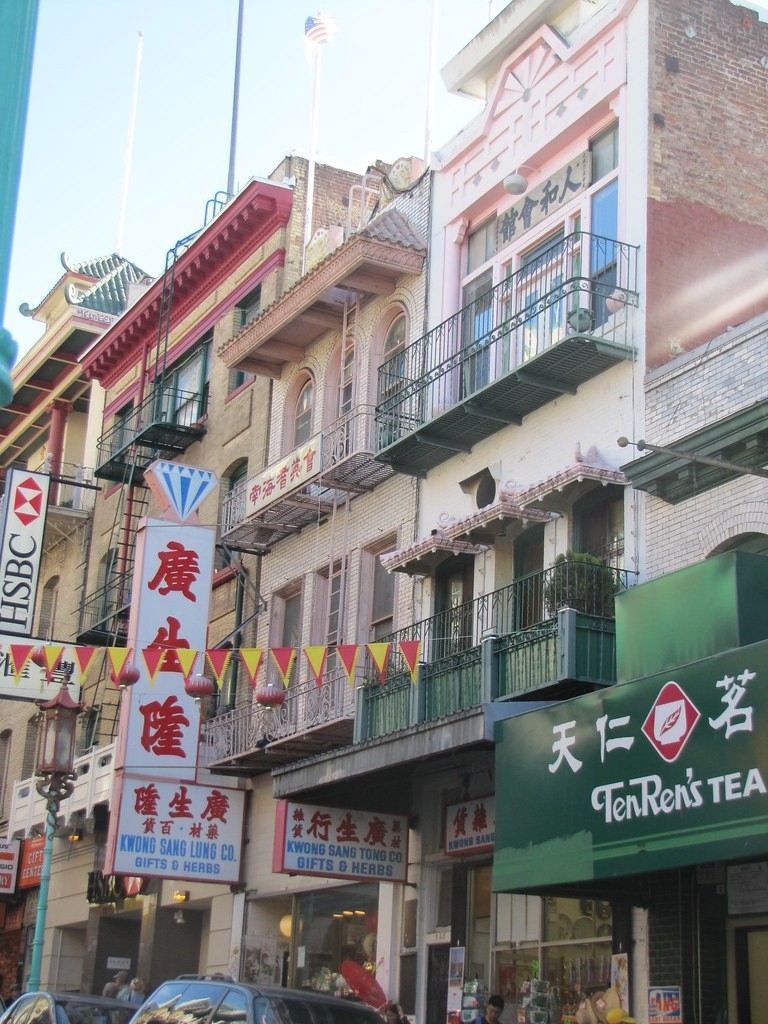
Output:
[341,957,387,1007]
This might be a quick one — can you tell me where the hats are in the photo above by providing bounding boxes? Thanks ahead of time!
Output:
[113,971,128,979]
[129,977,143,990]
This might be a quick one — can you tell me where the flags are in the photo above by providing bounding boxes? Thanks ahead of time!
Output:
[304,14,328,45]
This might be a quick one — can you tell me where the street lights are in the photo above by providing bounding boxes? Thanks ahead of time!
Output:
[25,671,87,994]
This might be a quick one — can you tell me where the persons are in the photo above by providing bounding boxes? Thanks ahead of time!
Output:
[4,985,22,1008]
[104,972,150,1006]
[453,995,504,1024]
[375,1002,411,1024]
[212,972,232,981]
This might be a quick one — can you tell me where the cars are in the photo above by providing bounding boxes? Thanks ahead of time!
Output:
[126,978,386,1024]
[0,990,143,1024]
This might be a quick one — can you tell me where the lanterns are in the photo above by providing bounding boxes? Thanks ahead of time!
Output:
[184,674,214,701]
[110,660,141,689]
[257,683,284,712]
[31,643,52,667]
